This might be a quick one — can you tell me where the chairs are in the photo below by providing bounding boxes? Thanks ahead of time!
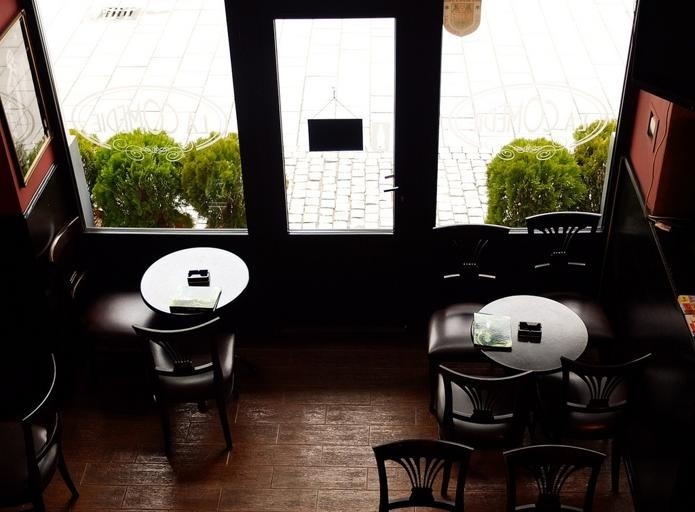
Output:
[1,353,80,512]
[132,317,236,457]
[428,211,653,496]
[502,445,606,511]
[371,439,474,512]
[48,215,159,409]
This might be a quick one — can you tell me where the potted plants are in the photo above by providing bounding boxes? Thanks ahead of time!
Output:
[69,126,245,229]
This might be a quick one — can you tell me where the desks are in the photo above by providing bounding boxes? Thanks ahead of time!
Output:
[140,246,250,317]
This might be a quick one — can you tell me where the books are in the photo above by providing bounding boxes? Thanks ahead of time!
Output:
[473,312,513,351]
[169,285,222,313]
